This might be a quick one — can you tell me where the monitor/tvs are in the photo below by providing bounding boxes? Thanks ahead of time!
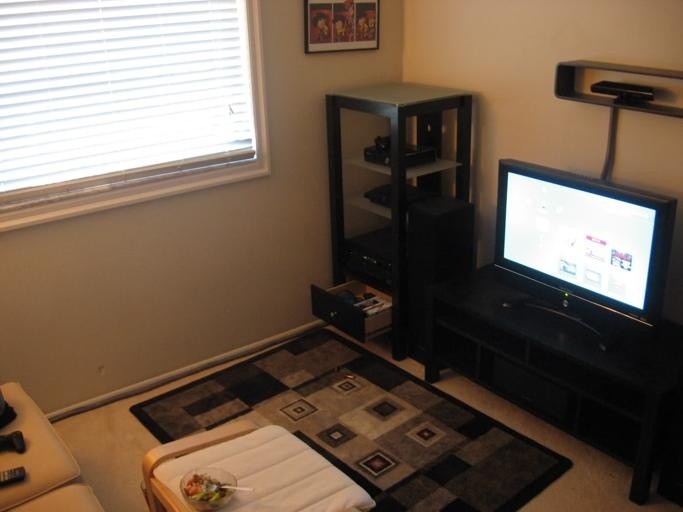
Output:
[492,159,679,350]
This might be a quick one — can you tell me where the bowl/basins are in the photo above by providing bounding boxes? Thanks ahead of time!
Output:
[180,467,237,512]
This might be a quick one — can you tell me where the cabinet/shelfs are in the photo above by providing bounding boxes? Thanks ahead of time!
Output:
[311,82,475,363]
[423,262,681,504]
[552,59,683,120]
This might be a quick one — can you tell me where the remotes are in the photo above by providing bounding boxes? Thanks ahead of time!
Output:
[0,466,26,489]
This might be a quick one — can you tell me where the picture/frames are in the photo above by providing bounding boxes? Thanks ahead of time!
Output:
[303,1,380,54]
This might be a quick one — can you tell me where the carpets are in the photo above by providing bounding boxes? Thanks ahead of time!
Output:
[129,330,574,511]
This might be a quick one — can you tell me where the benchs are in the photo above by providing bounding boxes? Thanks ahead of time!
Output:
[141,419,376,510]
